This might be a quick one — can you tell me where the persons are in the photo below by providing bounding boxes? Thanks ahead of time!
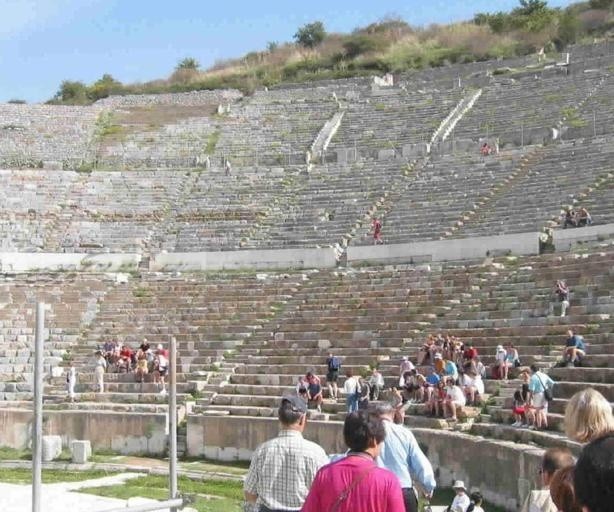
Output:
[446,481,469,512]
[244,395,330,512]
[66,361,77,403]
[370,217,383,246]
[480,142,488,158]
[521,448,587,512]
[300,410,405,512]
[576,207,592,228]
[563,208,577,230]
[553,279,571,318]
[564,388,614,512]
[464,492,484,512]
[550,465,589,512]
[293,327,586,432]
[92,333,170,395]
[342,402,437,512]
[489,140,498,157]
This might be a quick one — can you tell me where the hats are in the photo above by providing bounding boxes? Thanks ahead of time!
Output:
[453,481,466,491]
[282,395,308,414]
[435,353,441,358]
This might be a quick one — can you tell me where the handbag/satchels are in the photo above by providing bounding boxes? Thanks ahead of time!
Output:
[545,388,553,401]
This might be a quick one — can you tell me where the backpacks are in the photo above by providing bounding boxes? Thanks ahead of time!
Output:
[329,356,340,370]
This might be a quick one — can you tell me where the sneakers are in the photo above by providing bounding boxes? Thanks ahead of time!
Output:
[512,420,550,431]
